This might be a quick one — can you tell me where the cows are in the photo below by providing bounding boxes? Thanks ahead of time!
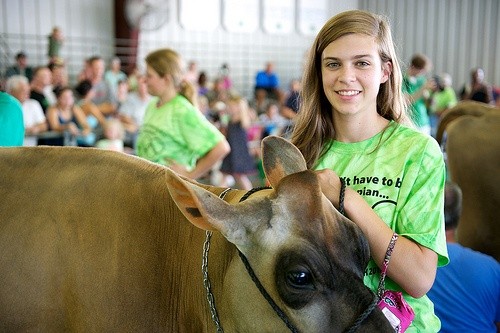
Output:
[437,100,500,263]
[1,135,394,333]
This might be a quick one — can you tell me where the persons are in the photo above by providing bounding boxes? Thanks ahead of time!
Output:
[135,49,231,180]
[178,58,302,193]
[425,182,500,333]
[0,26,152,156]
[459,67,495,106]
[289,10,451,333]
[426,74,458,140]
[0,75,31,147]
[399,52,437,136]
